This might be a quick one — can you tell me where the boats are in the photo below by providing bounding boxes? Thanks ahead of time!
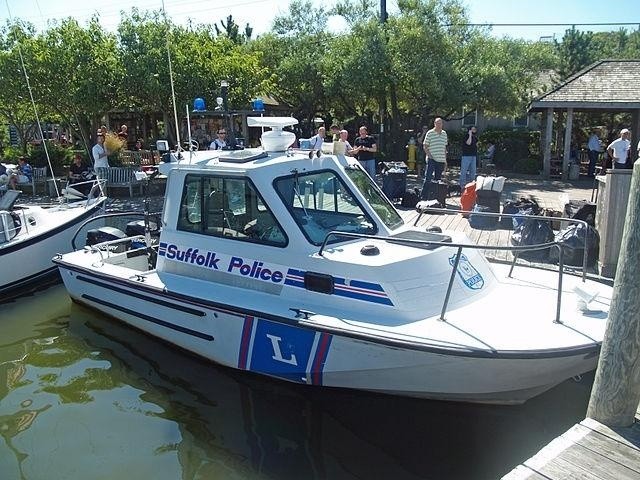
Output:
[0,42,112,306]
[48,1,614,414]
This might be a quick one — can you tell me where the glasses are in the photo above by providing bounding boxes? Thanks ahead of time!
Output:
[219,132,226,134]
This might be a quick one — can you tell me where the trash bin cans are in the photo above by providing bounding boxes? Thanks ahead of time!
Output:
[377,160,408,202]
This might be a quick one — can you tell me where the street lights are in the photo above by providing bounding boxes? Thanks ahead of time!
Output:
[221,80,229,111]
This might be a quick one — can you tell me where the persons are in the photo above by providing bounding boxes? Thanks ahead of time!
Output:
[570,128,640,176]
[418,118,448,195]
[69,154,91,193]
[460,127,478,191]
[181,123,261,150]
[484,141,495,159]
[310,125,377,181]
[0,156,33,202]
[88,126,111,199]
[118,125,129,141]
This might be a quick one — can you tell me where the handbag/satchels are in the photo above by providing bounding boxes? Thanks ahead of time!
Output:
[625,157,633,168]
[461,174,596,230]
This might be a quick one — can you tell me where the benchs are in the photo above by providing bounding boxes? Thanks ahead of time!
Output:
[9,167,47,196]
[96,166,144,197]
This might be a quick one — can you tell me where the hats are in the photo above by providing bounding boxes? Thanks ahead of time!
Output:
[591,128,601,132]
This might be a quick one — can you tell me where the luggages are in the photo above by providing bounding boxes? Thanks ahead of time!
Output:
[379,161,448,215]
